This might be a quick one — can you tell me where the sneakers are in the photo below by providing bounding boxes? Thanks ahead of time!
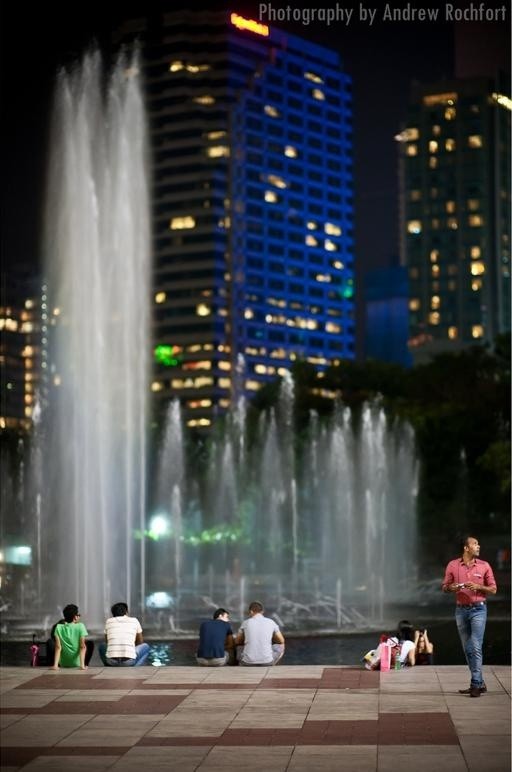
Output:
[460,687,486,697]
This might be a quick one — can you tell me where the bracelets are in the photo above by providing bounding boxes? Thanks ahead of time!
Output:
[446,586,451,592]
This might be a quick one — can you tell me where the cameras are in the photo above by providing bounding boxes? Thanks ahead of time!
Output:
[456,582,465,589]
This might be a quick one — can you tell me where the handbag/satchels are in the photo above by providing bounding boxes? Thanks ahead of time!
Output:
[365,635,400,670]
[32,640,53,665]
[413,631,432,654]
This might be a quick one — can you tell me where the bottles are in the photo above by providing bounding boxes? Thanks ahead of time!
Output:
[395,649,401,671]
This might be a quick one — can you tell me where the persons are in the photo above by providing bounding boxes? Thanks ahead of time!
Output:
[234,600,286,667]
[45,603,95,671]
[412,628,434,665]
[193,607,237,666]
[439,533,497,697]
[390,620,416,666]
[98,601,151,666]
